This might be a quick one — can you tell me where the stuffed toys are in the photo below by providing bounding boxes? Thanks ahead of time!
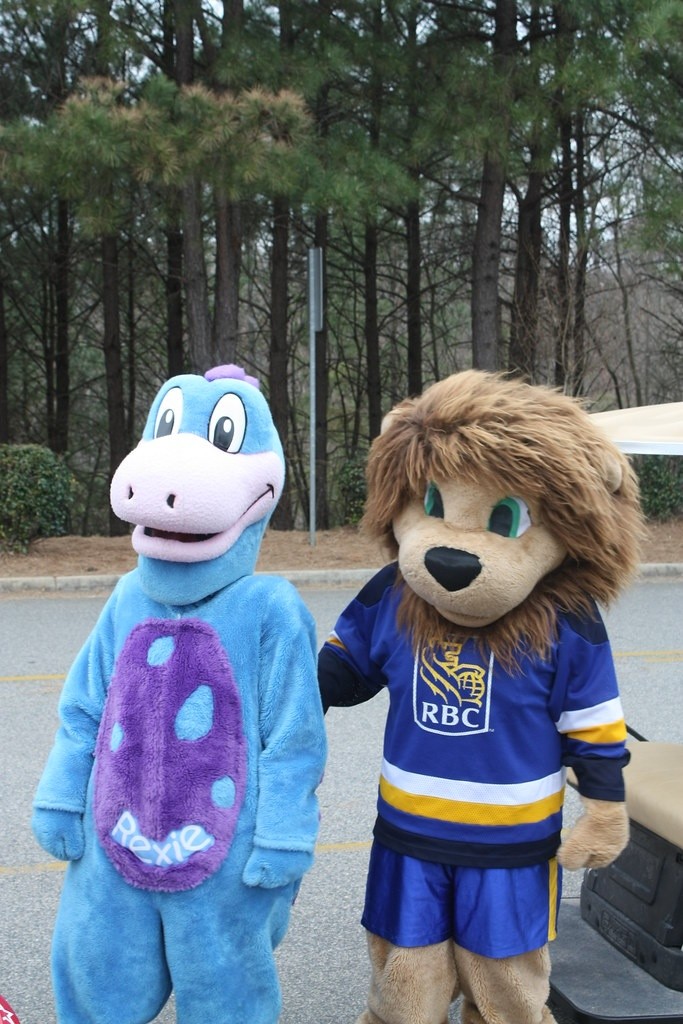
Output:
[318,364,636,1024]
[29,362,331,1023]
